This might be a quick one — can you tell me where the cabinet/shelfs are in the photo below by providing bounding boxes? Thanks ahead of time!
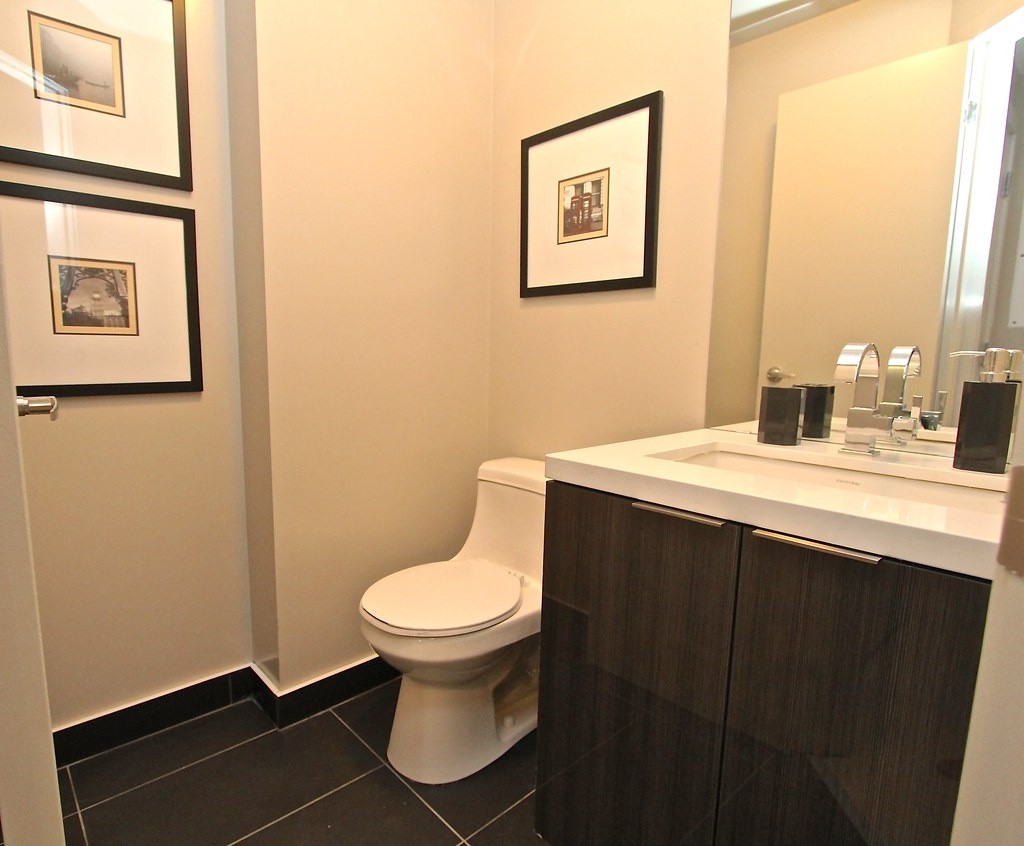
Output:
[528,476,995,846]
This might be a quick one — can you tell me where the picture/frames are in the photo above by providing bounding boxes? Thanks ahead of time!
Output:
[1,0,195,193]
[518,89,664,299]
[0,180,205,398]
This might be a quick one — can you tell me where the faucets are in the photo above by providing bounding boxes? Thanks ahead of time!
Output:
[831,341,924,461]
[874,344,948,436]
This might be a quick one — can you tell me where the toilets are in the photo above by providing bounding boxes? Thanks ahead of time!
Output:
[357,451,550,787]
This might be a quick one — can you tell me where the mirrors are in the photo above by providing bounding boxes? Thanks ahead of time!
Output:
[703,0,1024,493]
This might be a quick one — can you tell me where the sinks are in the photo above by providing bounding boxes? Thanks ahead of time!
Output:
[643,438,1010,525]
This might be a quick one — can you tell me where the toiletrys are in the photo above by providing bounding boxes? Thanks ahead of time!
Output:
[965,349,1023,468]
[949,347,1022,476]
